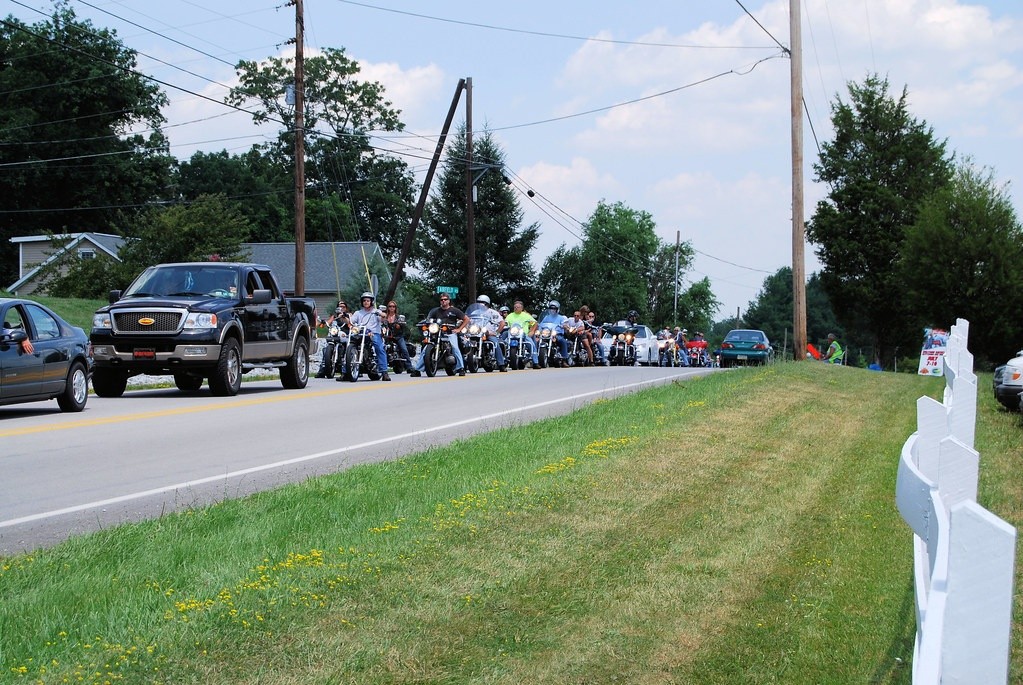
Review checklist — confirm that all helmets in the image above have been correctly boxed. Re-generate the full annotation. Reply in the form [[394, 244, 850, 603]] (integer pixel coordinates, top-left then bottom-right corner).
[[360, 292, 375, 303], [548, 300, 560, 314], [627, 310, 639, 319], [477, 295, 490, 305], [694, 331, 704, 338], [336, 300, 347, 308], [499, 306, 510, 313]]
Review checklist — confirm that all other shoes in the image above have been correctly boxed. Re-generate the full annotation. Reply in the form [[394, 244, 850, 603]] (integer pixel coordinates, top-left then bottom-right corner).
[[407, 367, 415, 373], [459, 369, 465, 376], [410, 370, 421, 377], [382, 373, 392, 381], [314, 373, 326, 379], [336, 373, 350, 381], [561, 361, 569, 368], [500, 365, 507, 372], [533, 364, 541, 369], [589, 360, 642, 366]]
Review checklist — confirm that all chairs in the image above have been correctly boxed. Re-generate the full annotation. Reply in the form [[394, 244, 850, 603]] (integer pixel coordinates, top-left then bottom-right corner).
[[830, 351, 846, 366], [732, 336, 740, 340], [752, 337, 759, 341]]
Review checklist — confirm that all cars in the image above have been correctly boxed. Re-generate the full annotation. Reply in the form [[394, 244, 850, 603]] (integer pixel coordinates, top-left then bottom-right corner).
[[720, 329, 773, 368], [601, 324, 663, 366], [0, 297, 97, 412], [993, 350, 1023, 414]]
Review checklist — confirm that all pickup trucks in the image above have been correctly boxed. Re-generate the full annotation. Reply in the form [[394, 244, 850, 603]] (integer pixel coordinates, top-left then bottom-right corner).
[[89, 262, 321, 396]]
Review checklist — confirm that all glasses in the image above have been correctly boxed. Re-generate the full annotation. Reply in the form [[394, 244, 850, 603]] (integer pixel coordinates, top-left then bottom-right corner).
[[389, 305, 396, 307], [574, 310, 594, 318], [440, 298, 448, 301], [674, 330, 679, 331], [665, 328, 670, 330], [630, 315, 636, 318]]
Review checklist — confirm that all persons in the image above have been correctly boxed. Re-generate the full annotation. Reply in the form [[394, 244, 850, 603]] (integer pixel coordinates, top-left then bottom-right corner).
[[336, 292, 391, 381], [822, 333, 843, 364], [314, 291, 353, 378], [869, 357, 883, 371], [410, 293, 469, 377], [381, 300, 414, 374], [499, 301, 541, 370], [606, 310, 641, 367], [538, 300, 569, 368], [2, 327, 34, 355], [565, 305, 606, 367], [659, 326, 706, 367], [471, 295, 507, 372]]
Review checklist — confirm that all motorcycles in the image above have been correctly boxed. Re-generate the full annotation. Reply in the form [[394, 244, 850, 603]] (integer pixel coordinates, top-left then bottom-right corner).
[[317, 312, 641, 382], [659, 338, 711, 367]]
